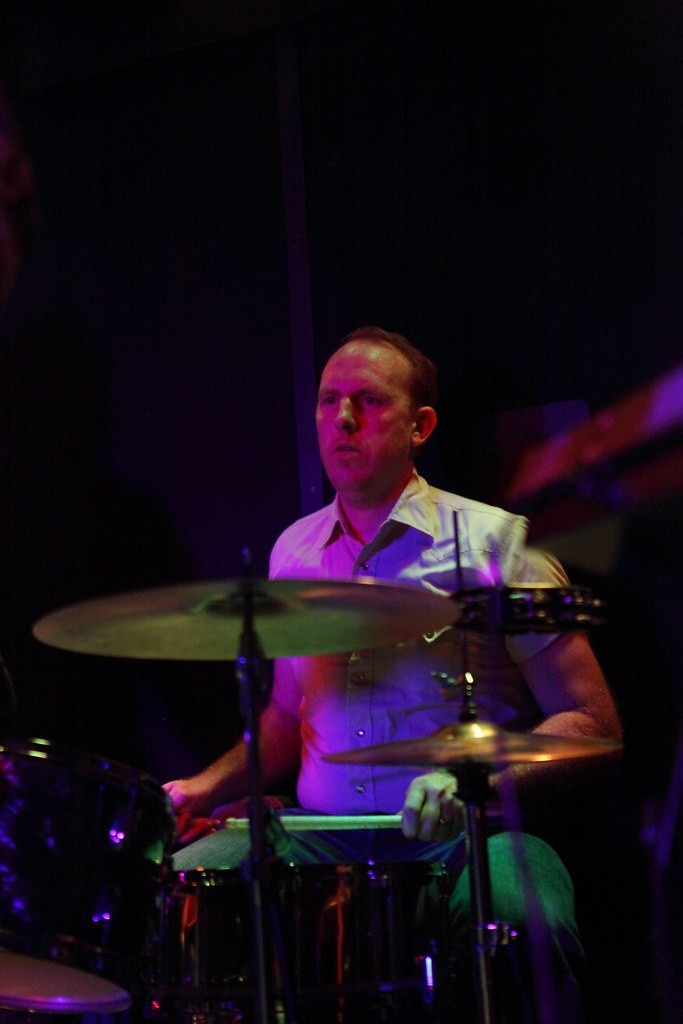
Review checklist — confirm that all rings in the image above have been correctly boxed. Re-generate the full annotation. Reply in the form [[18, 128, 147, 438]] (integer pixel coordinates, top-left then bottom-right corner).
[[438, 817, 453, 827]]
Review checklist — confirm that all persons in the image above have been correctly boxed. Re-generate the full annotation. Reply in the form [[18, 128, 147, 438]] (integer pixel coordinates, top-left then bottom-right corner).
[[159, 323, 629, 1023]]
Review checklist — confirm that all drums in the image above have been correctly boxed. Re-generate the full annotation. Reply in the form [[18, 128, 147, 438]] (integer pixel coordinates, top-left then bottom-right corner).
[[0, 736, 177, 1016], [452, 581, 610, 631], [152, 859, 451, 1024]]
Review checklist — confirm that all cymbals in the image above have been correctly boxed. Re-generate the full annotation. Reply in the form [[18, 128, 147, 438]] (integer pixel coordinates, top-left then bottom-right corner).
[[321, 718, 614, 769], [34, 576, 459, 660]]
